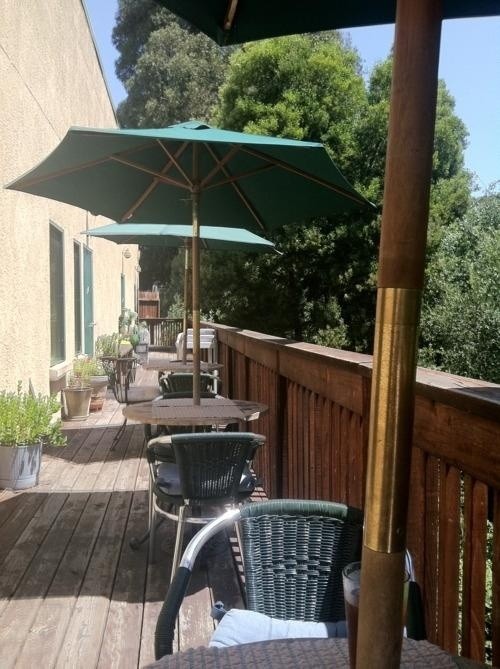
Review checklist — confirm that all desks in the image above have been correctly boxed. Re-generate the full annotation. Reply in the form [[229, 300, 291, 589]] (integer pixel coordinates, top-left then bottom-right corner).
[[140, 627, 488, 667]]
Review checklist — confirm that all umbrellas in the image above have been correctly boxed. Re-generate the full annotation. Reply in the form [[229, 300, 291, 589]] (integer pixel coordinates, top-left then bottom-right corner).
[[157, 0, 500, 667], [82, 224, 275, 366], [3, 117, 378, 406]]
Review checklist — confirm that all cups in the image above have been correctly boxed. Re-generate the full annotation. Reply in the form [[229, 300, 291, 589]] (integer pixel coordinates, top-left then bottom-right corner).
[[340, 560, 410, 669]]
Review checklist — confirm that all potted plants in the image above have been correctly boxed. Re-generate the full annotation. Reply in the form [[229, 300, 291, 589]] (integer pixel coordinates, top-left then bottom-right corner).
[[0, 383, 66, 491], [64, 359, 95, 421], [90, 359, 110, 413]]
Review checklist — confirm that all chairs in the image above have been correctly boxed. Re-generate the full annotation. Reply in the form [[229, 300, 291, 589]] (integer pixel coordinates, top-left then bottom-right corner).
[[155, 498, 427, 659]]
